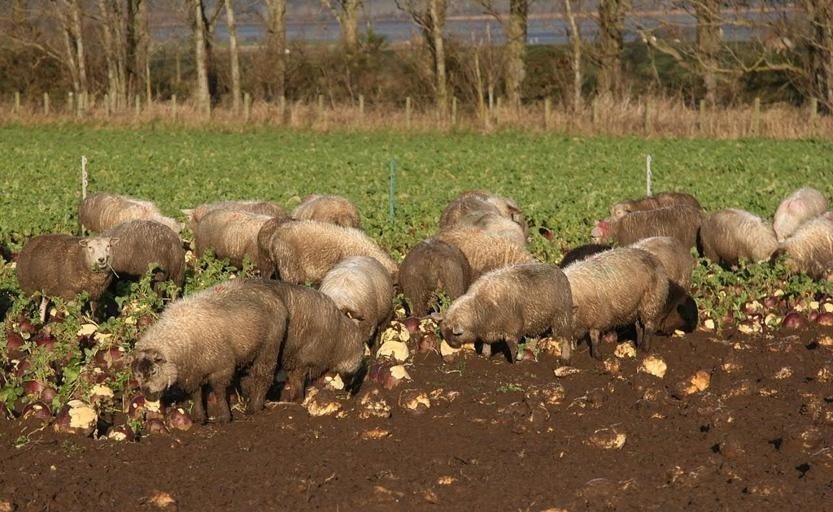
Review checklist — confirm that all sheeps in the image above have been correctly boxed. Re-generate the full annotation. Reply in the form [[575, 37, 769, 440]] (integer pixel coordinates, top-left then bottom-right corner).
[[15, 185, 833, 428]]
